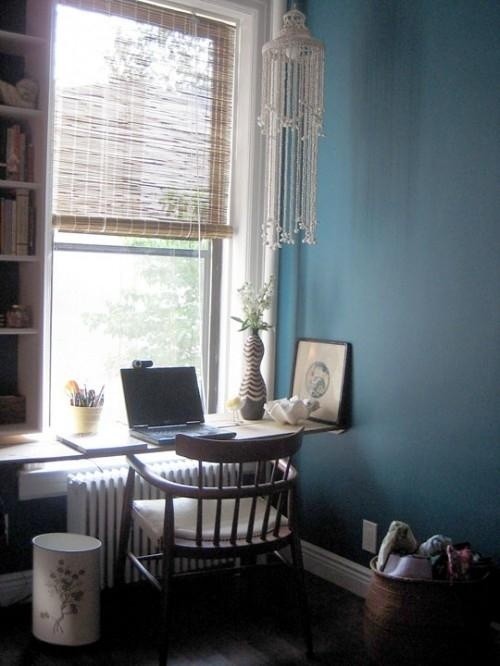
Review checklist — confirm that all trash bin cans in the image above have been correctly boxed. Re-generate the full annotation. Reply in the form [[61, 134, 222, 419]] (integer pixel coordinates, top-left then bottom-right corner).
[[31, 533, 103, 646]]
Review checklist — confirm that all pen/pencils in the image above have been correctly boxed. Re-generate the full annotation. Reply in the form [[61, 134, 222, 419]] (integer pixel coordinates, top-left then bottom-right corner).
[[64, 379, 105, 407]]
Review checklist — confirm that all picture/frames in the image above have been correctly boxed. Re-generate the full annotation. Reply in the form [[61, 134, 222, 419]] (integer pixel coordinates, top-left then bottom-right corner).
[[289, 338, 351, 425]]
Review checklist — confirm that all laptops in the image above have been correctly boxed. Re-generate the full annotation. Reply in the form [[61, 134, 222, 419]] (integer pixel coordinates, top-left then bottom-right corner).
[[119, 366, 237, 445]]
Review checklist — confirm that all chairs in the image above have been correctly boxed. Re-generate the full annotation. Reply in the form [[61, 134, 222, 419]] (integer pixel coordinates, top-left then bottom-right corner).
[[113, 426, 315, 666]]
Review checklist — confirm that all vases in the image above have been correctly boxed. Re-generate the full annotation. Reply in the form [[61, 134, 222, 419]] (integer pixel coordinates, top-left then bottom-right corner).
[[239, 329, 267, 420]]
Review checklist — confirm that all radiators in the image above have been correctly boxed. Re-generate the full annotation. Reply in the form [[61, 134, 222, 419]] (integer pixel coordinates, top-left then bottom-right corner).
[[67, 461, 258, 589]]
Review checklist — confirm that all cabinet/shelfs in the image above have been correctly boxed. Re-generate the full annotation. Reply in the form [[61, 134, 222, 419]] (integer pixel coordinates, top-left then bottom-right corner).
[[0, 1, 55, 447]]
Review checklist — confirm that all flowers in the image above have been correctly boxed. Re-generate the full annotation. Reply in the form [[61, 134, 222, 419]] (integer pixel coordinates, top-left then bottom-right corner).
[[231, 273, 278, 339]]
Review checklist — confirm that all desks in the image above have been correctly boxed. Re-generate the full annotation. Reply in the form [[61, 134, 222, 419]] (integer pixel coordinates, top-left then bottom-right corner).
[[1, 413, 348, 466]]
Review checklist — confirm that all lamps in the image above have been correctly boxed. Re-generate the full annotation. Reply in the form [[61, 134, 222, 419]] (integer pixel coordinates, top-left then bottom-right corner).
[[256, 0, 325, 250]]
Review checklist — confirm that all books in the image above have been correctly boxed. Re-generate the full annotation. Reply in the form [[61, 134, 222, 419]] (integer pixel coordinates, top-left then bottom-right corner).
[[0, 123, 36, 256], [56, 429, 148, 455]]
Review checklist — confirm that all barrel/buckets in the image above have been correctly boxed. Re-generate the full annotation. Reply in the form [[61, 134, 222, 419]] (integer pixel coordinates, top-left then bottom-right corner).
[[361, 560, 484, 664]]
